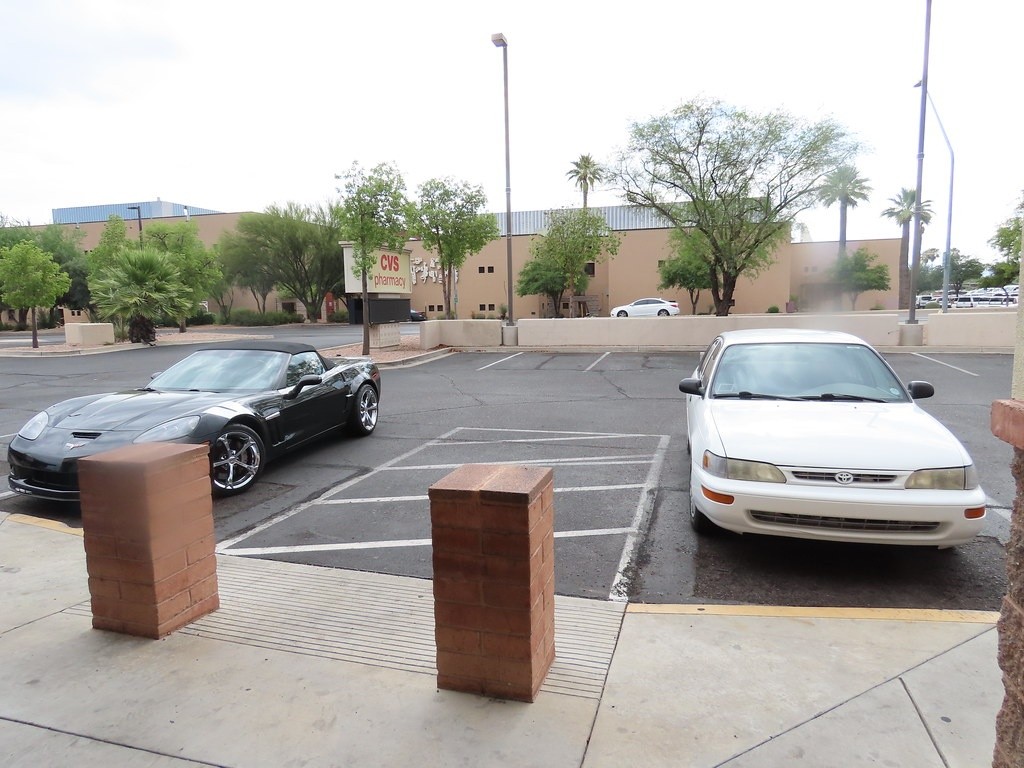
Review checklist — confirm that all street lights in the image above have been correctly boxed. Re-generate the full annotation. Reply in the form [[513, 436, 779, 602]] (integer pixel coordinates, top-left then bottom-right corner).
[[491, 32, 513, 326], [127, 206, 143, 249]]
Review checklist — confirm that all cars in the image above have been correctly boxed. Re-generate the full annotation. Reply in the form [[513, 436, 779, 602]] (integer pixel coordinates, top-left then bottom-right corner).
[[610, 297, 680, 318], [678, 327, 987, 550], [410, 309, 428, 322], [915, 284, 1020, 309]]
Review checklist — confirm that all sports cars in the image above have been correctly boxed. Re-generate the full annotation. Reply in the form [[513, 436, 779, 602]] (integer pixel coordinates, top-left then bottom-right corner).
[[7, 339, 381, 502]]
